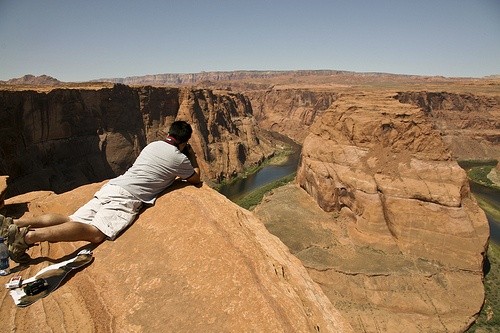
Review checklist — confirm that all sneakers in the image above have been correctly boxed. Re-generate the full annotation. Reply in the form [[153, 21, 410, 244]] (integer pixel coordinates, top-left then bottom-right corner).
[[7, 223, 31, 266], [0, 215, 15, 245]]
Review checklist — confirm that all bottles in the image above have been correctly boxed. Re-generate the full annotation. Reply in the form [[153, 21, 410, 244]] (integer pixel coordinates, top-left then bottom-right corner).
[[0, 237, 11, 276]]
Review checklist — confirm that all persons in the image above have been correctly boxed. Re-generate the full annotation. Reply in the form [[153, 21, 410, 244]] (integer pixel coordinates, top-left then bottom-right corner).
[[0, 120, 200, 264]]
